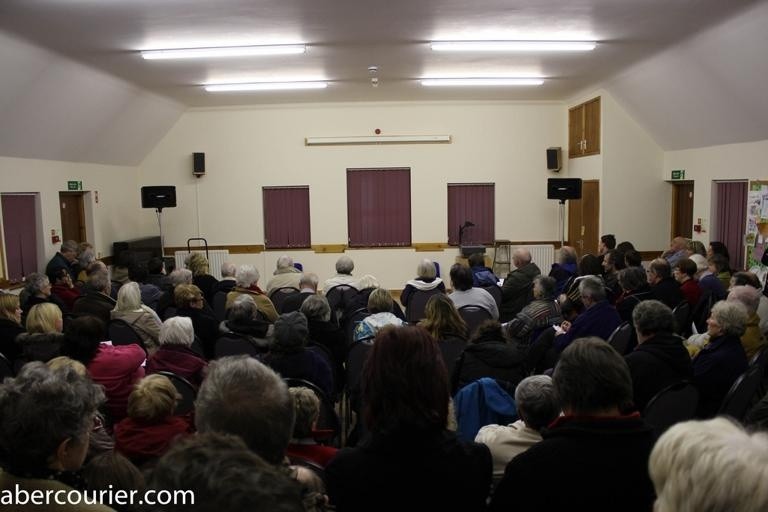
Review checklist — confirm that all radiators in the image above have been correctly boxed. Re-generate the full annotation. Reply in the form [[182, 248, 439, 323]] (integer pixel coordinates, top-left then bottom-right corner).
[[175, 249, 229, 280], [500, 244, 556, 278]]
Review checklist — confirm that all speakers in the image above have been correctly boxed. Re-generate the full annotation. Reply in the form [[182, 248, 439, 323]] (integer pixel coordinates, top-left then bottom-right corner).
[[141, 186, 176, 208], [546, 147, 561, 170], [192, 152, 205, 174], [547, 178, 582, 199]]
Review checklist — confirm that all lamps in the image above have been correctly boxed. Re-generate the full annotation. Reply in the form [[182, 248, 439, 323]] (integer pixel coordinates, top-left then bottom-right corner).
[[458, 220, 475, 246]]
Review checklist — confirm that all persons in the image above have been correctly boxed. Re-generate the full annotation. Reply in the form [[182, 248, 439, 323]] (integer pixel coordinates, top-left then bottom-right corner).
[[0, 236, 768, 512]]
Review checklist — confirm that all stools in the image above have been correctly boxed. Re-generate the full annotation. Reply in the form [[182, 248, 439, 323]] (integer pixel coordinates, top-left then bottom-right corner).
[[491, 240, 512, 273]]
